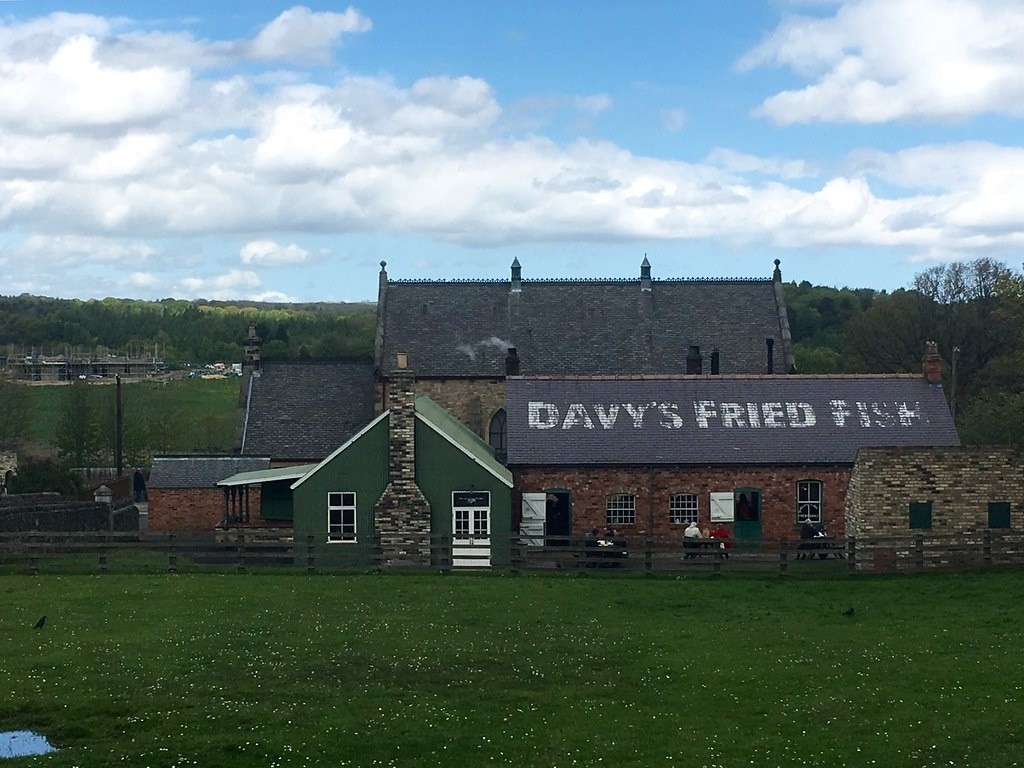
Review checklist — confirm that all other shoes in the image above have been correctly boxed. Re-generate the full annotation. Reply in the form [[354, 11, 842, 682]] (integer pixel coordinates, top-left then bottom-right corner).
[[720, 555, 724, 559], [684, 556, 688, 559], [585, 562, 622, 568], [725, 553, 729, 559]]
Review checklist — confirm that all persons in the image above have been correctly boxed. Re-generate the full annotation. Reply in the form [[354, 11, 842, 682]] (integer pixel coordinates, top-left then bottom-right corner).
[[683, 521, 703, 559], [709, 523, 733, 559], [2, 470, 20, 495], [736, 494, 752, 521], [582, 526, 629, 568], [797, 518, 820, 558]]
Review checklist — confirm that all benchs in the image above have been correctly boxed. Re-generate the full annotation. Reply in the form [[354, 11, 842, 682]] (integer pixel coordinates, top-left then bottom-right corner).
[[682, 536, 729, 560], [800, 536, 845, 561]]
